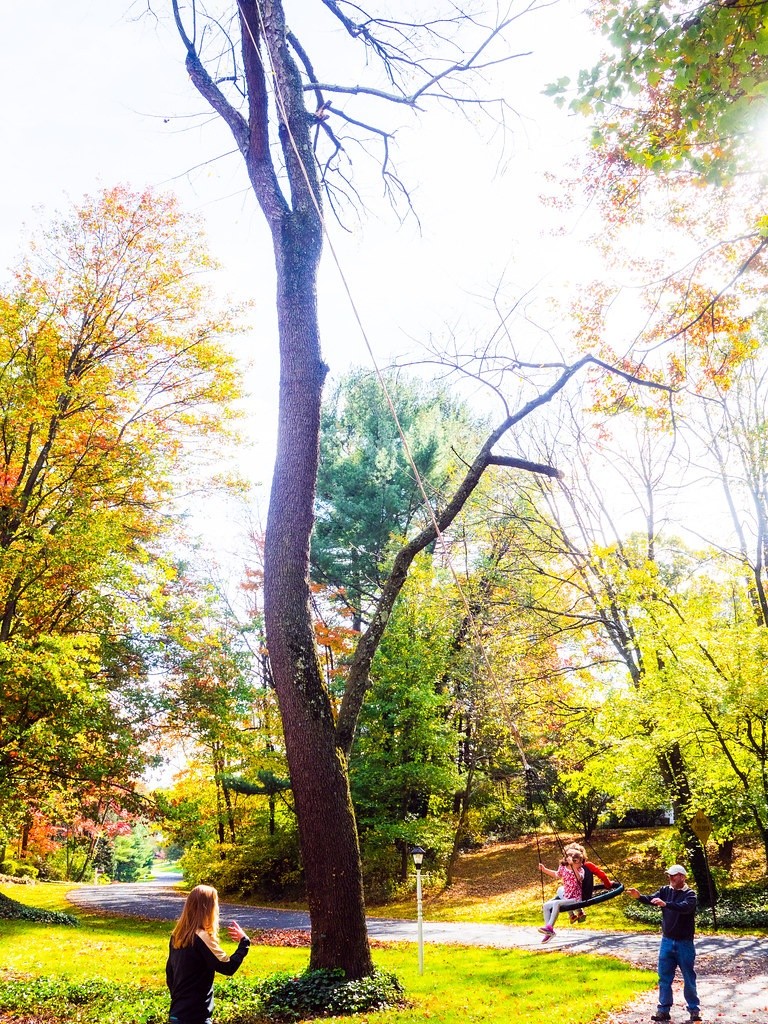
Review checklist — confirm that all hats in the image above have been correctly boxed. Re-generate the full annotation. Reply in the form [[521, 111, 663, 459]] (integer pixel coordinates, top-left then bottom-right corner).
[[664, 865, 686, 877]]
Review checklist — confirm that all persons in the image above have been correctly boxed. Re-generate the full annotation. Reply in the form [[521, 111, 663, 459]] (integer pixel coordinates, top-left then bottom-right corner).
[[538, 842, 615, 944], [166, 885, 251, 1024], [625, 864, 701, 1021]]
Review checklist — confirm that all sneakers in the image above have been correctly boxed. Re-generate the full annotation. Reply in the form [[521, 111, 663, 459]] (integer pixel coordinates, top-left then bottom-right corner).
[[538, 927, 552, 936], [651, 1011, 671, 1021], [569, 915, 577, 924], [690, 1012, 702, 1021], [577, 912, 586, 924], [542, 930, 557, 944]]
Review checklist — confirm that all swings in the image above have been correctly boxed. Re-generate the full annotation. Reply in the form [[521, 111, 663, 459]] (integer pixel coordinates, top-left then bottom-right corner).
[[524, 766, 625, 913]]
